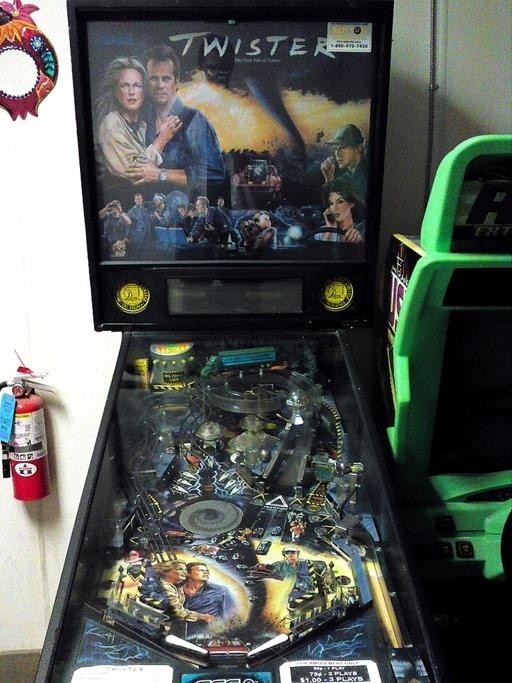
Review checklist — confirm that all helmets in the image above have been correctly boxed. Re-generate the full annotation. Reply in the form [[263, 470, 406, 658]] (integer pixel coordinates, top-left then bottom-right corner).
[[282, 547, 299, 554]]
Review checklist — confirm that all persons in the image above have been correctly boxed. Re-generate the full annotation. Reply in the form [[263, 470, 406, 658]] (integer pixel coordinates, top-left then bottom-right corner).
[[121, 542, 314, 640]]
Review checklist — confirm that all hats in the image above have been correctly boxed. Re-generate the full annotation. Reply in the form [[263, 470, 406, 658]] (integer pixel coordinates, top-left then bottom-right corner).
[[326, 123, 363, 148]]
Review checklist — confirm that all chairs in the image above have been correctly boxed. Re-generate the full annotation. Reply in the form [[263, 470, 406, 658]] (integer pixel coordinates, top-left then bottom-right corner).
[[386, 132, 512, 579]]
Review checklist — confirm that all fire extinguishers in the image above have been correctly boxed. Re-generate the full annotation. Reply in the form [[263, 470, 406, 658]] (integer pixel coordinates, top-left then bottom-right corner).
[[0, 367, 58, 501]]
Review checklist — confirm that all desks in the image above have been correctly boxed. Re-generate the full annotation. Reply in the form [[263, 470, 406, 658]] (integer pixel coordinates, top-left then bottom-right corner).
[[33, 333, 441, 683]]
